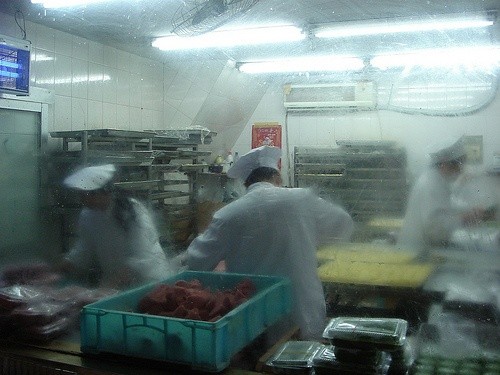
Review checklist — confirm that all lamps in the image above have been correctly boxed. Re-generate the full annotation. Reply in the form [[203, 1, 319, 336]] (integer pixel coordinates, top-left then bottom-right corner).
[[153, 6, 500, 74]]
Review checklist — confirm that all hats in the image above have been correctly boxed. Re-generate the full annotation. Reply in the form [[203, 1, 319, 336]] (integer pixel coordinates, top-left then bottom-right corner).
[[427, 138, 465, 164], [63, 163, 116, 191], [226, 145, 281, 183]]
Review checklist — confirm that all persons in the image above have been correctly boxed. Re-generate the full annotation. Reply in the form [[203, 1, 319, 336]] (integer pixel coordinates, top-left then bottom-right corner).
[[174, 149, 354, 338], [404, 137, 500, 248], [61, 163, 169, 288]]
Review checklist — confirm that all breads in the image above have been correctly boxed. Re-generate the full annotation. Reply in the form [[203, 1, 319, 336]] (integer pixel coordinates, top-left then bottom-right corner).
[[136, 280, 255, 323]]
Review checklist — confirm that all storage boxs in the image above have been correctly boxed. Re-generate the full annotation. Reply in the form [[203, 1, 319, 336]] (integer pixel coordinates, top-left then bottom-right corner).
[[81, 268, 292, 371]]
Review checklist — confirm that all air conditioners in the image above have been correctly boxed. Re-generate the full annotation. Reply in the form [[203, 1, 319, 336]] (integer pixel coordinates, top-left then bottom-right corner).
[[284, 83, 376, 112]]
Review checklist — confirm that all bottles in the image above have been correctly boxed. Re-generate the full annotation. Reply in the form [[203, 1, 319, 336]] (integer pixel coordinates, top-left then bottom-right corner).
[[199, 151, 239, 173]]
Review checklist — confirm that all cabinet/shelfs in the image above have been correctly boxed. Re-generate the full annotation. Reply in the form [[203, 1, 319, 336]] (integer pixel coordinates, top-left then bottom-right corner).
[[62, 133, 199, 257]]
